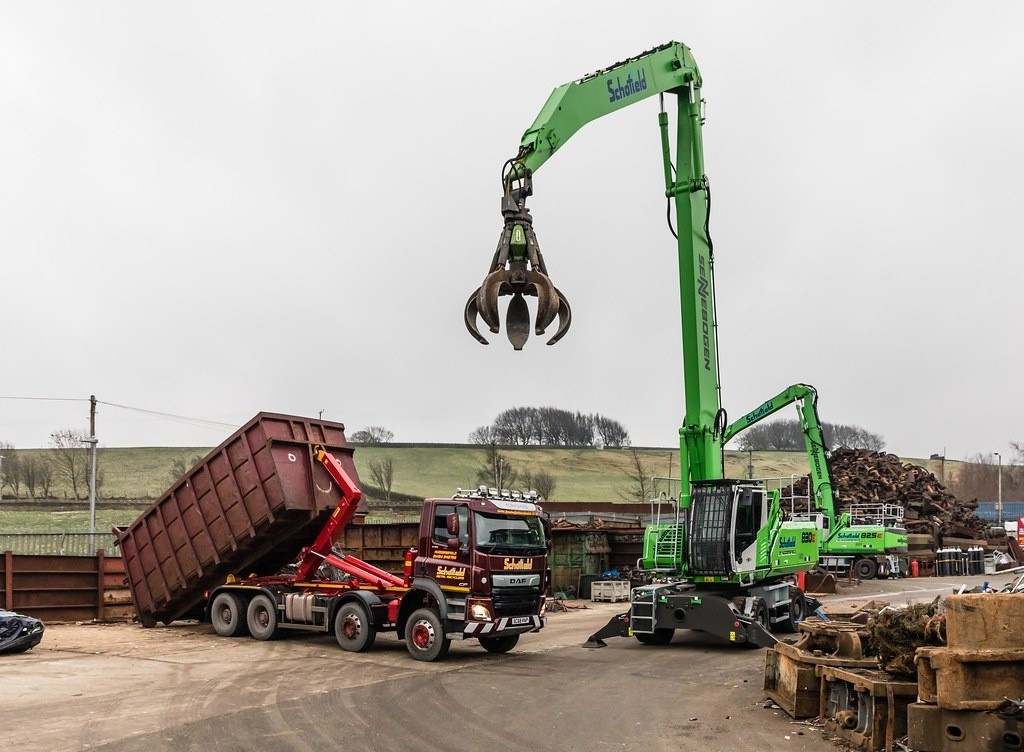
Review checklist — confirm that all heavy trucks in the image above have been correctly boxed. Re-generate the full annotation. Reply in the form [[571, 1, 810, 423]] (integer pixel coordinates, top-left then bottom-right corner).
[[112, 409, 552, 661]]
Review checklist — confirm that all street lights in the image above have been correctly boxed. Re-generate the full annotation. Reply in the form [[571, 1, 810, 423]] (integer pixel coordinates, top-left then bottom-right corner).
[[993, 453, 1001, 525]]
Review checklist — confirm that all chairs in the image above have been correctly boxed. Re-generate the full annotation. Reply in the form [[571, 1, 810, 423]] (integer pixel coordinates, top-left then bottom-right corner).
[[735, 505, 752, 537]]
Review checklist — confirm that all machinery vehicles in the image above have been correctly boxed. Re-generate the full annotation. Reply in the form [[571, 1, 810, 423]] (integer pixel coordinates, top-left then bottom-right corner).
[[723, 384, 909, 581], [462, 39, 852, 650]]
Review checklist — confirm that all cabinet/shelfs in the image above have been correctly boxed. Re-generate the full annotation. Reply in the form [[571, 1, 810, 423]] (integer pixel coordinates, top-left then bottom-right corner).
[[591, 581, 630, 602]]
